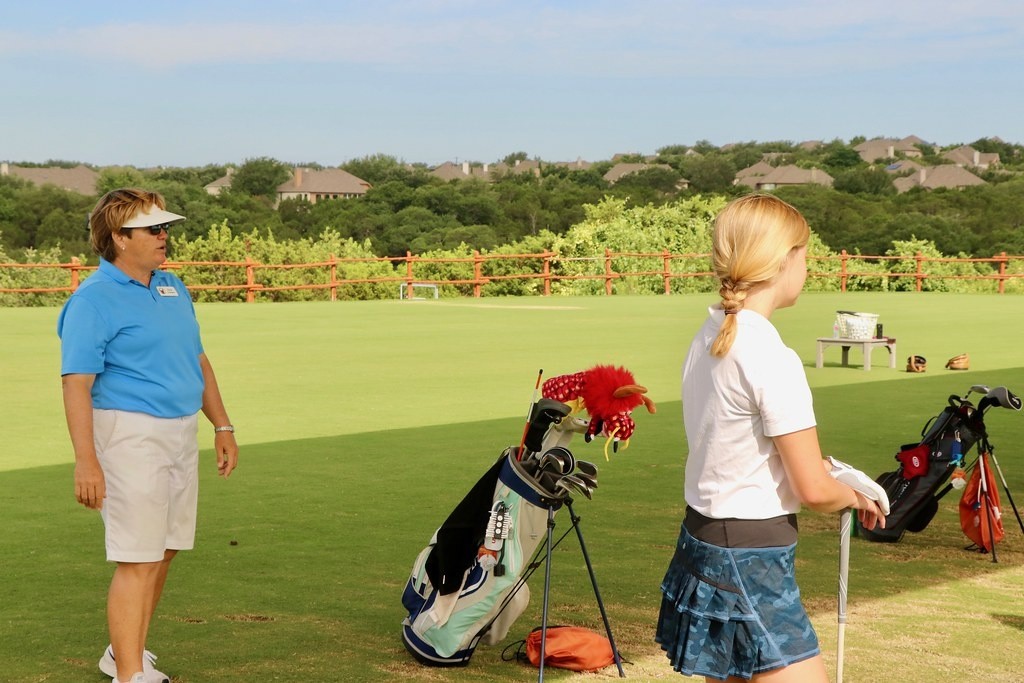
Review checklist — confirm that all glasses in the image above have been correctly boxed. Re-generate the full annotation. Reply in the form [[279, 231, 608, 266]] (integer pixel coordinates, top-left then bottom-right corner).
[[127, 223, 170, 235]]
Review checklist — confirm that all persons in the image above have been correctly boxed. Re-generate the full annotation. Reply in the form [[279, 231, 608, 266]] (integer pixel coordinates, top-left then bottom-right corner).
[[57, 188, 239, 683], [654, 195, 890, 683]]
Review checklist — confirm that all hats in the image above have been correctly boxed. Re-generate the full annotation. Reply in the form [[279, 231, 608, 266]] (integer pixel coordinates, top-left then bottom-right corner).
[[122, 204, 185, 228]]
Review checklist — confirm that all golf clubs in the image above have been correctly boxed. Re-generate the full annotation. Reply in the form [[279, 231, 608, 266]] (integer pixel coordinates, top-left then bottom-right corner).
[[948, 384, 990, 413], [835, 506, 855, 683], [553, 459, 600, 501]]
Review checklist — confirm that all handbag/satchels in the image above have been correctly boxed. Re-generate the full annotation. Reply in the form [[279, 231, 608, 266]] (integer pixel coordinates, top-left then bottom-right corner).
[[907, 356, 926, 372], [502, 625, 620, 674], [826, 455, 890, 516], [946, 353, 968, 370], [959, 436, 1004, 554]]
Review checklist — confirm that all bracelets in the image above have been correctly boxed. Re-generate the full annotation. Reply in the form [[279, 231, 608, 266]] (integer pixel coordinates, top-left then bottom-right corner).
[[215, 425, 234, 433]]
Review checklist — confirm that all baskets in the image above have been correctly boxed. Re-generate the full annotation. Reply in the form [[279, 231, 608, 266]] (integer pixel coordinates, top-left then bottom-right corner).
[[846, 317, 869, 340], [838, 311, 879, 340]]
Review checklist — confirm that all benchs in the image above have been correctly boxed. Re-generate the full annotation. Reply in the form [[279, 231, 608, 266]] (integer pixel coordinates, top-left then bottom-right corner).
[[400, 284, 439, 298]]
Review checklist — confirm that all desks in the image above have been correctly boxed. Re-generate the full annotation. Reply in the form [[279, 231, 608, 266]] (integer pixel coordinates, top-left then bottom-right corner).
[[816, 337, 897, 371]]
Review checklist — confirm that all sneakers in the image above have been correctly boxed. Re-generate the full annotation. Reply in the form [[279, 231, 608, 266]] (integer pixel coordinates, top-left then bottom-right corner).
[[99, 643, 170, 683], [112, 671, 149, 683]]
